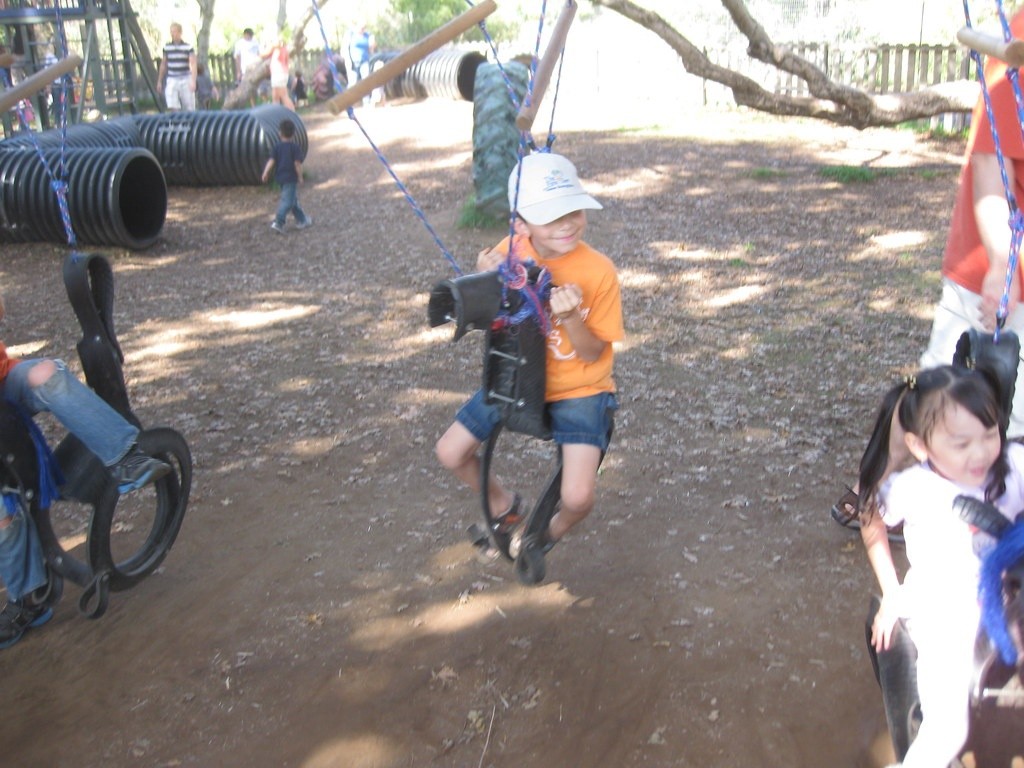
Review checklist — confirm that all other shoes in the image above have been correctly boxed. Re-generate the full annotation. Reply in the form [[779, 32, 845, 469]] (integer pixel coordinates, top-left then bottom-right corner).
[[295, 214, 313, 230], [271, 220, 289, 236]]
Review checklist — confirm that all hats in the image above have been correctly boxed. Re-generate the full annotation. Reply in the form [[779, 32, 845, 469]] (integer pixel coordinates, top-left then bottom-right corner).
[[506, 152, 603, 227]]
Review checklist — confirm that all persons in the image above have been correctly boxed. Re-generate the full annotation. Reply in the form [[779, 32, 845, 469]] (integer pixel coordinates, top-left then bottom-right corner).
[[262, 120, 313, 233], [857, 367, 1024, 768], [235, 28, 296, 113], [195, 65, 219, 111], [156, 23, 198, 112], [1, 339, 170, 649], [434, 153, 624, 559], [1, 30, 61, 130], [291, 71, 308, 109], [925, 5, 1024, 439], [344, 24, 373, 108]]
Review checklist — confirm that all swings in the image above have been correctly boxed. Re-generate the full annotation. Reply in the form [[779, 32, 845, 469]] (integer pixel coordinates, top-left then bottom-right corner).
[[862, 0, 1023, 768], [0, 1, 196, 619], [312, 0, 617, 587]]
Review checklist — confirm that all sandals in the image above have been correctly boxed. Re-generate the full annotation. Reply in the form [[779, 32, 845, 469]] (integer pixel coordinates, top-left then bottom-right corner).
[[476, 491, 529, 562], [508, 508, 570, 561], [829, 482, 905, 544]]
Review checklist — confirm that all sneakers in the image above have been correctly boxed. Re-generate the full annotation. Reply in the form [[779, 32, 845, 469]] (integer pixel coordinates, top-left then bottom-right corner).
[[106, 437, 173, 496], [0, 591, 52, 649]]
